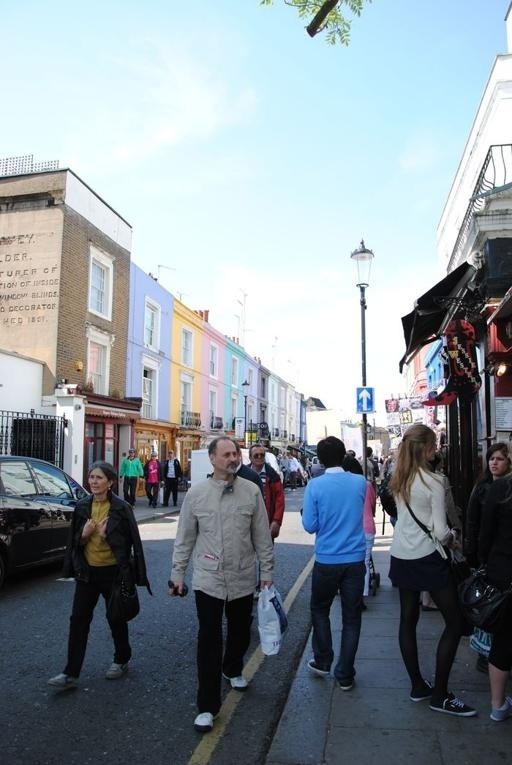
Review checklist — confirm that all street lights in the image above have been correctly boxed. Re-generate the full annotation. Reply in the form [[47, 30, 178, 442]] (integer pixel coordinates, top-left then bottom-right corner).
[[240, 378, 251, 443], [349, 238, 377, 490]]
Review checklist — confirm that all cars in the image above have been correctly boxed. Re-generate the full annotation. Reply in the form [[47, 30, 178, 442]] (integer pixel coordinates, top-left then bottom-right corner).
[[278, 455, 325, 489], [0, 453, 91, 583]]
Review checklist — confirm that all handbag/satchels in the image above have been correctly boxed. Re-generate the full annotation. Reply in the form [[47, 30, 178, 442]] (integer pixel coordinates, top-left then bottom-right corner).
[[377, 472, 397, 520], [106, 567, 140, 625], [455, 563, 512, 629], [256, 584, 290, 656]]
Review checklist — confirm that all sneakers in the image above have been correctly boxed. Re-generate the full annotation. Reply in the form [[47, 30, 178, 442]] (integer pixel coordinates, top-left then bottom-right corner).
[[410, 679, 435, 702], [490, 695, 512, 722], [335, 672, 354, 690], [222, 669, 249, 690], [308, 657, 330, 675], [194, 710, 214, 731], [429, 689, 477, 717], [104, 663, 129, 679], [48, 672, 81, 689]]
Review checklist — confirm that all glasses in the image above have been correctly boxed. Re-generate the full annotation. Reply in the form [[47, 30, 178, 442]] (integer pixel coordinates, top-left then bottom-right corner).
[[252, 453, 264, 459]]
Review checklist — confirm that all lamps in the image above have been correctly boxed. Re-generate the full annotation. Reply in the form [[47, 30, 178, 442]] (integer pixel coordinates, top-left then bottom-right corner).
[[74, 360, 84, 372]]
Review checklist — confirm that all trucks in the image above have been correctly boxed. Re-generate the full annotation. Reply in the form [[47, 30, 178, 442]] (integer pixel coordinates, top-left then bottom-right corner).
[[188, 447, 286, 513]]
